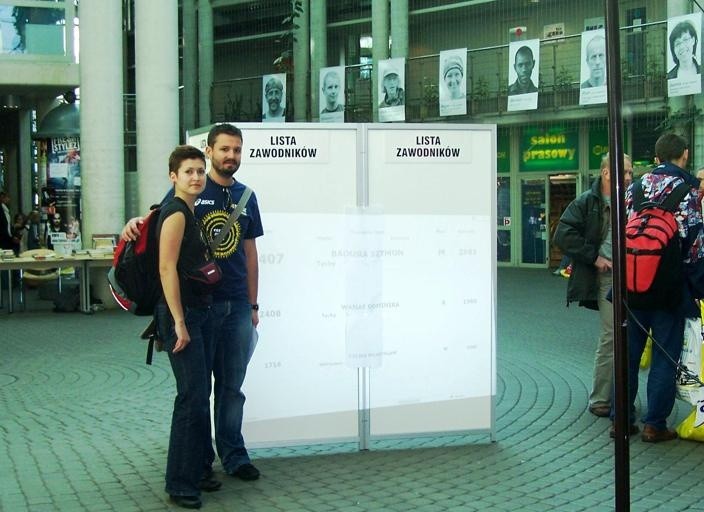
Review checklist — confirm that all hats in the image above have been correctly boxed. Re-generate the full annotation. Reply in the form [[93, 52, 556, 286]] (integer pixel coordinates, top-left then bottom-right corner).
[[265, 78, 283, 92], [383, 66, 399, 78], [442, 55, 464, 79]]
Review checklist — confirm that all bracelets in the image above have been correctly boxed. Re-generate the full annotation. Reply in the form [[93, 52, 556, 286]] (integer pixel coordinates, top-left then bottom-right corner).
[[251, 304, 259, 310]]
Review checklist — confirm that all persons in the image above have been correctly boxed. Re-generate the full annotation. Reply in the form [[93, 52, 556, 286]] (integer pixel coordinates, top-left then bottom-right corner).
[[439, 55, 465, 100], [605, 134, 704, 444], [122, 125, 264, 481], [581, 35, 607, 88], [0, 192, 68, 258], [666, 21, 701, 80], [153, 145, 221, 509], [379, 65, 405, 108], [508, 46, 538, 95], [554, 152, 633, 418], [321, 71, 344, 114], [262, 78, 286, 119]]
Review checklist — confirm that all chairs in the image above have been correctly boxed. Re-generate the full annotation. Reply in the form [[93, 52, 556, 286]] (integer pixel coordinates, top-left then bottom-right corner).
[[19, 249, 61, 311]]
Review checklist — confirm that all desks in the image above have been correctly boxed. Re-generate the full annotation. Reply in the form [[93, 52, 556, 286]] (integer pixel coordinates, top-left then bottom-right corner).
[[87, 256, 115, 313], [0, 255, 86, 314]]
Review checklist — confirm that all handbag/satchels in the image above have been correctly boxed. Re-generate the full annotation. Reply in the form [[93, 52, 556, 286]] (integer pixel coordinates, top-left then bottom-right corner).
[[183, 257, 225, 296]]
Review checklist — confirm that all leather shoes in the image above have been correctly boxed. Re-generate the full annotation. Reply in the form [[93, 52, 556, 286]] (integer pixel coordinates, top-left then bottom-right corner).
[[589, 407, 610, 417], [610, 423, 639, 438], [169, 494, 201, 509], [641, 425, 678, 443], [237, 463, 259, 481], [204, 479, 221, 493]]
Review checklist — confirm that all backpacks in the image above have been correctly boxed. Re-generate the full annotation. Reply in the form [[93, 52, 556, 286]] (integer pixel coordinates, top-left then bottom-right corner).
[[625, 177, 698, 312], [107, 200, 193, 366]]
